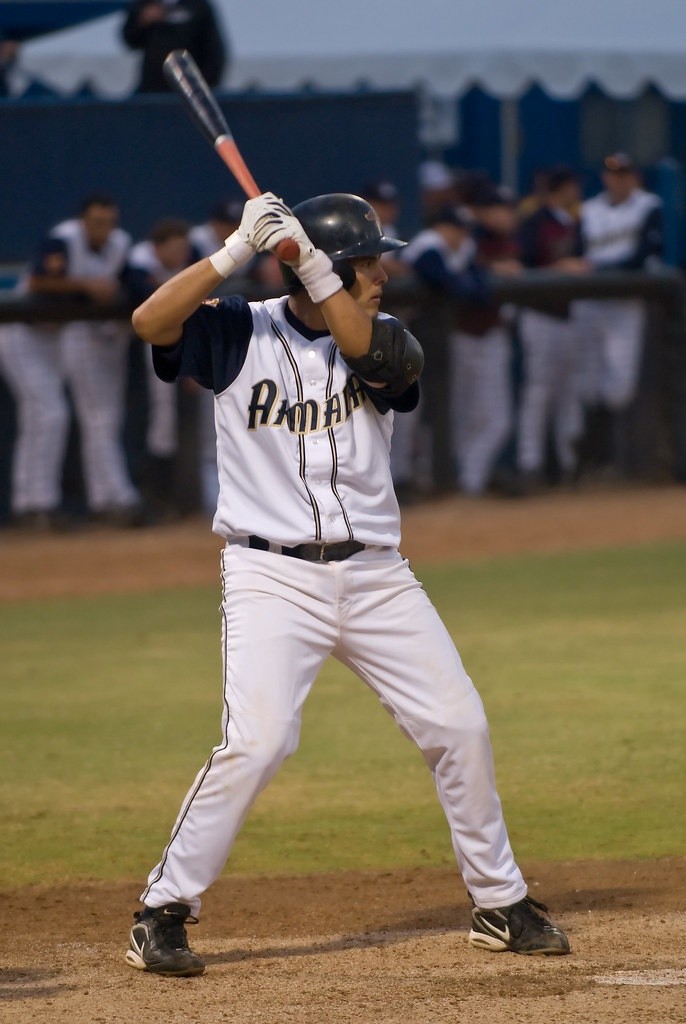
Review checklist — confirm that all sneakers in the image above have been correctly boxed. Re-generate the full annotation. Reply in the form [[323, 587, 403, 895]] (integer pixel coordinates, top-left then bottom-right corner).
[[464, 889, 570, 954], [123, 904, 205, 977]]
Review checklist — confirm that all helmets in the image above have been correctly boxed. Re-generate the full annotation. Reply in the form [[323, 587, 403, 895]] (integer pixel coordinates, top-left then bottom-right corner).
[[277, 192, 408, 293]]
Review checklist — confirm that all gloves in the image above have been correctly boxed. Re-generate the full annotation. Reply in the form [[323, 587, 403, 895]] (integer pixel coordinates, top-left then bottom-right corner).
[[210, 192, 294, 279], [246, 208, 343, 303]]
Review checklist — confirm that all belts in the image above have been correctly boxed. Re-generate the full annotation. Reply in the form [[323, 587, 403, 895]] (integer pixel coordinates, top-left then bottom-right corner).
[[249, 535, 365, 565]]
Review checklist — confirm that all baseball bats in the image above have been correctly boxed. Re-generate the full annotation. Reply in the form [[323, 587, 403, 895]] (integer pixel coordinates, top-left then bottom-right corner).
[[162, 48, 300, 262]]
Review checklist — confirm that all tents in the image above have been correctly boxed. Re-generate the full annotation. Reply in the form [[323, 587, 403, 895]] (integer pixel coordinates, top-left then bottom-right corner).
[[1, 1, 686, 323]]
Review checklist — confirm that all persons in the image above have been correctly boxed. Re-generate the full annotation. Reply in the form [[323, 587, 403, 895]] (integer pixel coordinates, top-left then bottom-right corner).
[[0, 155, 662, 525], [124, 196, 570, 978]]
